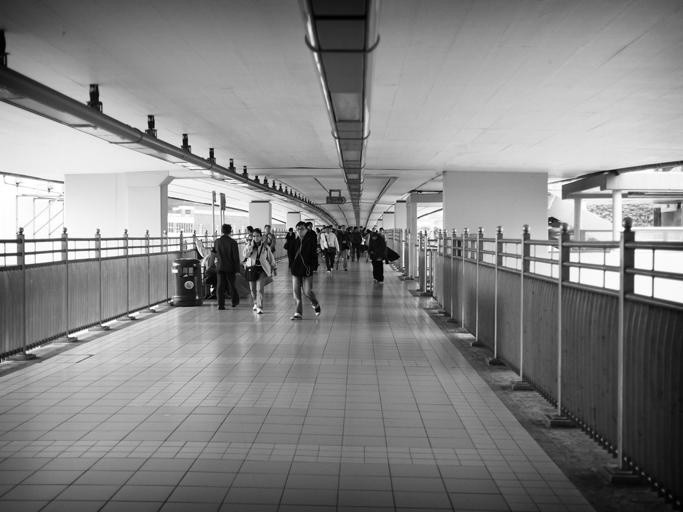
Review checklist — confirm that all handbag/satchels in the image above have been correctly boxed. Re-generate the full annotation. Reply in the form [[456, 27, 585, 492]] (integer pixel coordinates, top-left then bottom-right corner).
[[245, 265, 260, 280], [328, 247, 336, 254]]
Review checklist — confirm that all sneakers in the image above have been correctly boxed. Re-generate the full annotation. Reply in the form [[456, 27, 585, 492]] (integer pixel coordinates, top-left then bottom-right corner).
[[253, 304, 264, 314], [290, 306, 321, 320]]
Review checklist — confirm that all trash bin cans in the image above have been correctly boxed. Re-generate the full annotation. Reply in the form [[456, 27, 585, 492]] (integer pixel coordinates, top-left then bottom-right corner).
[[170, 258, 203, 306]]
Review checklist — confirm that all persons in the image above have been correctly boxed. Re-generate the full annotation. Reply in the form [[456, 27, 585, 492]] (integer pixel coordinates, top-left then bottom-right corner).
[[244, 223, 400, 286], [212, 223, 241, 311], [241, 227, 277, 316], [200, 248, 221, 299], [288, 221, 321, 321]]
